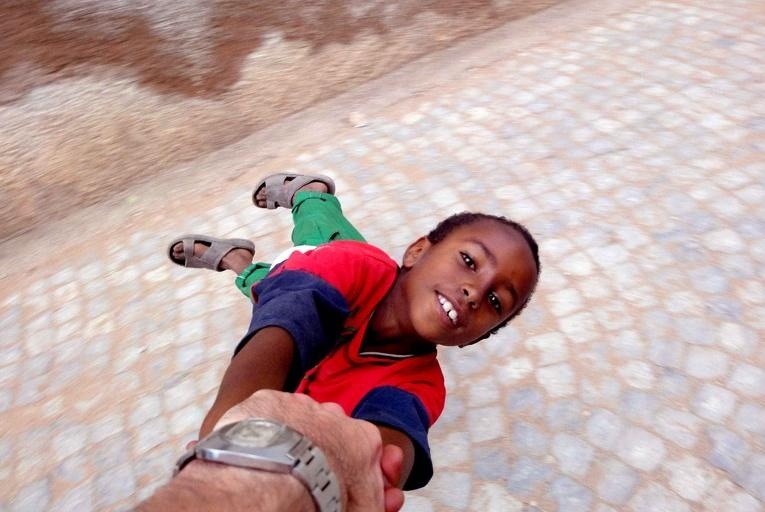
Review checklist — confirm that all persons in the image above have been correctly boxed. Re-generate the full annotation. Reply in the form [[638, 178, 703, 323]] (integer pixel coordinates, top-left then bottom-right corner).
[[166, 167, 543, 512], [121, 386, 394, 512]]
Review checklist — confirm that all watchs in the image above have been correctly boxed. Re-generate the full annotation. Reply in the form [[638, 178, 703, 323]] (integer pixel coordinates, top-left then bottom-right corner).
[[169, 415, 345, 512]]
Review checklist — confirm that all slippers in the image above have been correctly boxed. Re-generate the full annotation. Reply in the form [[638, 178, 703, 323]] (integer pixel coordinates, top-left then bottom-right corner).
[[252, 172, 336, 210], [167, 235, 256, 273]]
